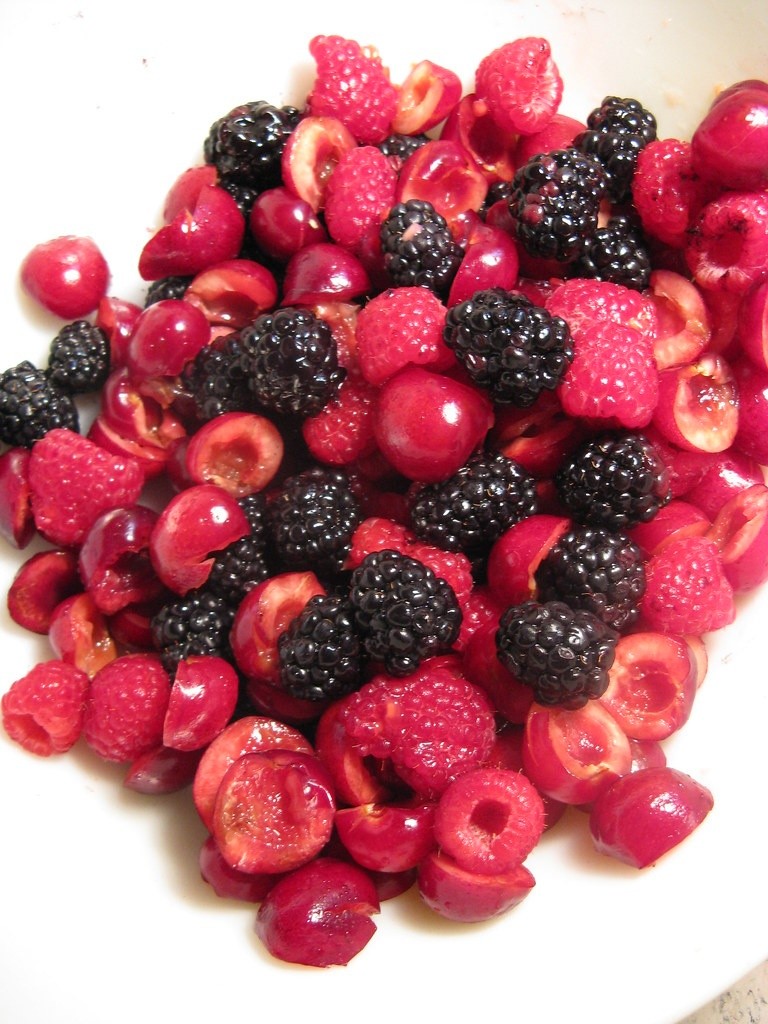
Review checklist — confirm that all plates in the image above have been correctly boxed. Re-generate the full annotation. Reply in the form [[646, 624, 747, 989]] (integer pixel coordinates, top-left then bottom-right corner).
[[3, 2, 768, 1023]]
